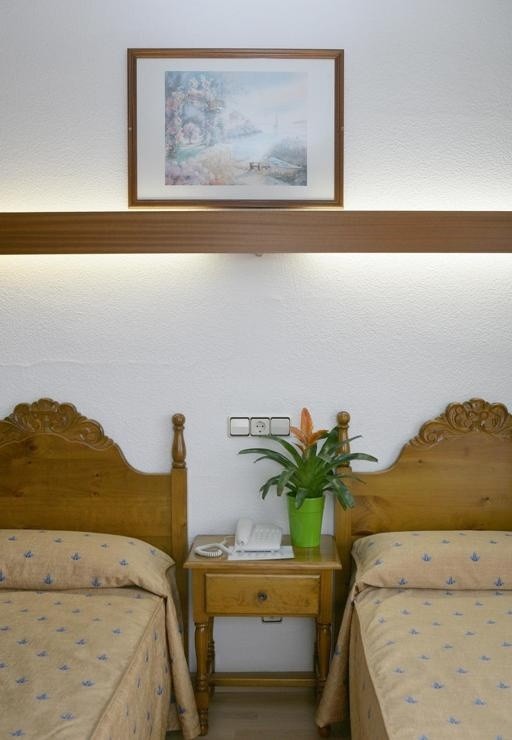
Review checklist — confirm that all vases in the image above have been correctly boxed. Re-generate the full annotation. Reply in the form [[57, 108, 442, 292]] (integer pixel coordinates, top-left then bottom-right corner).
[[287, 491, 326, 547]]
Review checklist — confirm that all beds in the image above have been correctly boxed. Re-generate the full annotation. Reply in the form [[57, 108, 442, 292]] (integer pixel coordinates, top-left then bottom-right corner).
[[0, 396, 188, 740], [336, 398, 512, 740]]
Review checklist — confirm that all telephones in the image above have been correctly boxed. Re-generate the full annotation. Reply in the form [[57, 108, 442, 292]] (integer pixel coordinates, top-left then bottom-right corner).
[[235, 519, 282, 551]]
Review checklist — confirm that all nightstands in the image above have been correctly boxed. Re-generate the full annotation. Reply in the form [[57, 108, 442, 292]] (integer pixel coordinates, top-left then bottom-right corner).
[[183, 535, 343, 736]]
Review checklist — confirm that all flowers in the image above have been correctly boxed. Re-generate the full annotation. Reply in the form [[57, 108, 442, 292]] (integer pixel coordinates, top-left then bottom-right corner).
[[237, 408, 378, 511]]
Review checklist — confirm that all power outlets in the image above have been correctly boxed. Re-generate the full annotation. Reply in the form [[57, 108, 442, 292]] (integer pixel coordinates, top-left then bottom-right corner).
[[250, 417, 270, 438]]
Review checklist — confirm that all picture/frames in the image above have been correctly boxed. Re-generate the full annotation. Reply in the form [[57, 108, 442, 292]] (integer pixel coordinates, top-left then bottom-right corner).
[[125, 47, 345, 209]]
